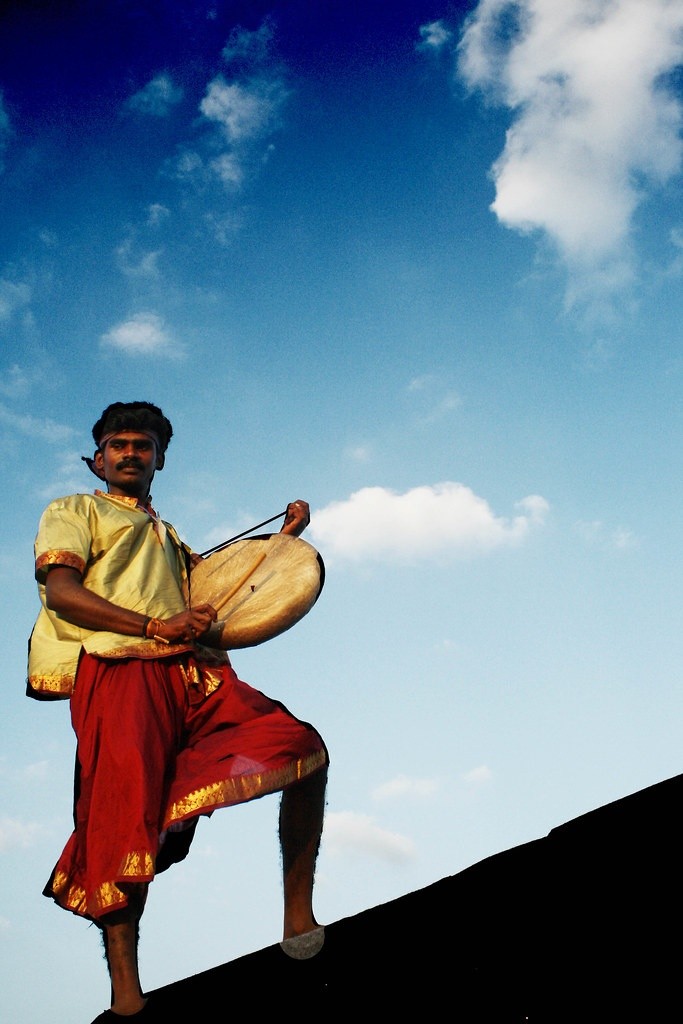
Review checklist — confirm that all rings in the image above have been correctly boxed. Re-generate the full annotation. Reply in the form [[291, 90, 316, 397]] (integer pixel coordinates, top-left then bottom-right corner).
[[295, 503, 301, 506]]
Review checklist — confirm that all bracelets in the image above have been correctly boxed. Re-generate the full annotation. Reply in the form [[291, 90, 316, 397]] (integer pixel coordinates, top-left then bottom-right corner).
[[143, 617, 170, 644]]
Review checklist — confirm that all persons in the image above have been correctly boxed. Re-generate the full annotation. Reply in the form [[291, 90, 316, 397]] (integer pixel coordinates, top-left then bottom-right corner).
[[27, 401, 330, 1023]]
